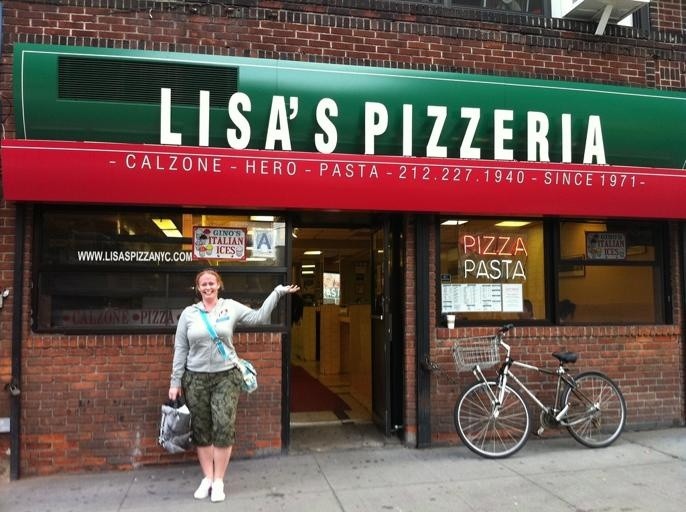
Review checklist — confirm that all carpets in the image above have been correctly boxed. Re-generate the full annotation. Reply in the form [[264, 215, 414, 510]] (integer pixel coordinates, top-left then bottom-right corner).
[[291, 364, 352, 413]]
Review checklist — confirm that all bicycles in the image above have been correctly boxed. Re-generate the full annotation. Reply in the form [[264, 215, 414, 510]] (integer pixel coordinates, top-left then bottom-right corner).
[[449, 322, 626, 459]]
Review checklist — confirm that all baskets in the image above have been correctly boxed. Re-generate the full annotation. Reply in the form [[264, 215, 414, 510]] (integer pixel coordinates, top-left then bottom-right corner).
[[451, 335, 501, 373]]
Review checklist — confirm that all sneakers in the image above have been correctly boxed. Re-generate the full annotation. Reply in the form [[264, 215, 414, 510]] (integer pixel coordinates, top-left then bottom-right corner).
[[194, 476, 212, 499], [211, 483, 225, 501]]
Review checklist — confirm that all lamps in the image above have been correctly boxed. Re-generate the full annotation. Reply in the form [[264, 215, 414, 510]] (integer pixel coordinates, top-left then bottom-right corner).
[[292, 228, 305, 238]]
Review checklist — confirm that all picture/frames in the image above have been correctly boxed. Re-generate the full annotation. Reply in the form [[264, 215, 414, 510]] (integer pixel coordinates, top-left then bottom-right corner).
[[559, 253, 585, 278], [627, 245, 647, 256]]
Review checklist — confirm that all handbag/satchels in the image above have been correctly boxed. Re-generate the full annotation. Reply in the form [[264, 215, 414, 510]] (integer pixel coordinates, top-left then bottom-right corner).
[[159, 397, 194, 455], [231, 358, 258, 394]]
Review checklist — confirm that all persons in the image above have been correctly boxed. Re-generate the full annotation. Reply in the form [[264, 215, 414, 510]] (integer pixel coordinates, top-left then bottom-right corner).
[[517, 299, 538, 320], [167, 268, 301, 501], [559, 298, 577, 323]]
